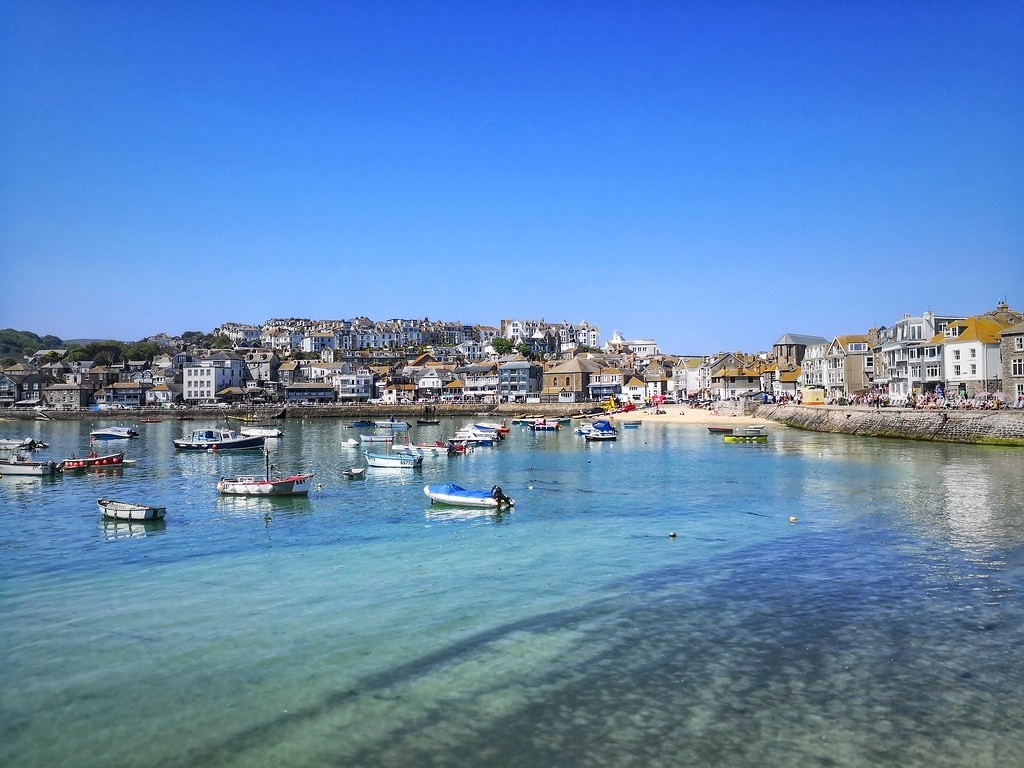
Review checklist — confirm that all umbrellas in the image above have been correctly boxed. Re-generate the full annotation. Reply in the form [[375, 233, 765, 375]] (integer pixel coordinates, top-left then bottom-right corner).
[[976, 391, 991, 404], [991, 392, 1009, 410]]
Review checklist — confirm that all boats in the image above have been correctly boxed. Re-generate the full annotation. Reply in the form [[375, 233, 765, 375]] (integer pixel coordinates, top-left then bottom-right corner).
[[90, 425, 140, 440], [423, 482, 516, 513], [171, 410, 270, 453], [240, 424, 286, 438], [0, 435, 51, 460], [177, 415, 194, 420], [140, 417, 162, 423], [227, 413, 262, 422], [342, 402, 667, 480], [216, 447, 316, 497], [62, 450, 127, 470], [97, 497, 167, 521], [0, 446, 63, 476], [709, 423, 767, 444]]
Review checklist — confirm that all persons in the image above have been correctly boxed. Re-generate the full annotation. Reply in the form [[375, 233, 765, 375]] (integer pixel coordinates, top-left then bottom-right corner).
[[825, 389, 1009, 410], [493, 486, 514, 513], [776, 392, 803, 405]]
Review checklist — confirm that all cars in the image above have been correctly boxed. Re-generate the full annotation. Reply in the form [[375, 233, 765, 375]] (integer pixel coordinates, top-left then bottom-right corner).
[[33, 404, 46, 412], [161, 401, 311, 409], [88, 403, 134, 412]]
